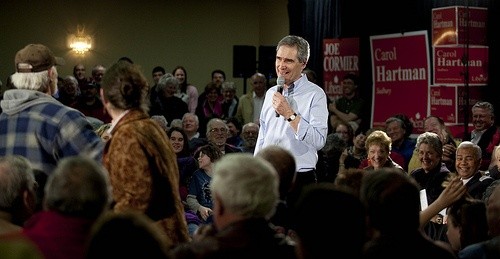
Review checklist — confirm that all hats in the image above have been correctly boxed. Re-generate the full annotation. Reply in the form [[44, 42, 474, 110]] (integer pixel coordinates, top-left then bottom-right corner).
[[15, 43, 66, 73]]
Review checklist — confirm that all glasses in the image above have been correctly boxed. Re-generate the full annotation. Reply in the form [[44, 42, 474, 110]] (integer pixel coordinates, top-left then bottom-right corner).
[[208, 129, 228, 133]]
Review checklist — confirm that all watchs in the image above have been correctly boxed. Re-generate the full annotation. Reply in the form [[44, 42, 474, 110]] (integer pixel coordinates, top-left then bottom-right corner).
[[287, 112, 298, 121]]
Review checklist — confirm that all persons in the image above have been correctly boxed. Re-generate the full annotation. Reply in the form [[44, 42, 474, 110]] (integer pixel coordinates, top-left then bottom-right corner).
[[253, 35, 329, 188], [0, 45, 500, 259]]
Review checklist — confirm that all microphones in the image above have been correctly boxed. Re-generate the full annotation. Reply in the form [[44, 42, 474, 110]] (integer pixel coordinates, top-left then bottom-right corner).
[[275, 76, 284, 116]]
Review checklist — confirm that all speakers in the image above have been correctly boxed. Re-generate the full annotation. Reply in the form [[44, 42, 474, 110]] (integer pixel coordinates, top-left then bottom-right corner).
[[232, 44, 258, 77], [259, 44, 278, 78]]
